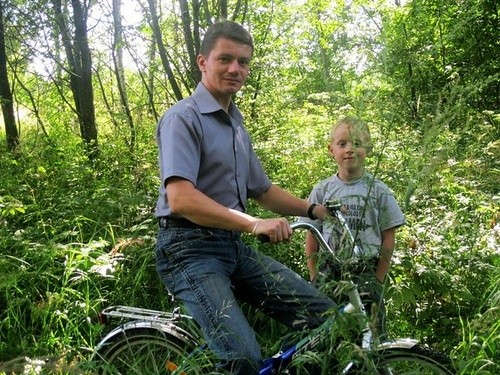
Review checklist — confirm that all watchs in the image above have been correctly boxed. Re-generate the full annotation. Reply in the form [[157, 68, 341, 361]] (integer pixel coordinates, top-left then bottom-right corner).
[[308, 203, 321, 220]]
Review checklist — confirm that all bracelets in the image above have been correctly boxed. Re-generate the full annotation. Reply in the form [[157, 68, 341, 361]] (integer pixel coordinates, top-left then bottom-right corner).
[[252, 219, 260, 237]]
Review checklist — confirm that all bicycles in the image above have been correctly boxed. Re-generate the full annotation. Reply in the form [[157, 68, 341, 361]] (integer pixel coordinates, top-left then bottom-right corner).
[[88, 198, 457, 375]]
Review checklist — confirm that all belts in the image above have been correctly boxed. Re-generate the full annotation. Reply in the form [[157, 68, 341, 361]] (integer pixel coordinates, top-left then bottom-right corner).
[[159, 216, 206, 228]]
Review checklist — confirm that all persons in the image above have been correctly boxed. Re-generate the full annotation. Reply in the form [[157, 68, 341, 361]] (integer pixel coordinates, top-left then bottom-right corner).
[[152, 21, 345, 375], [305, 119, 405, 375]]
